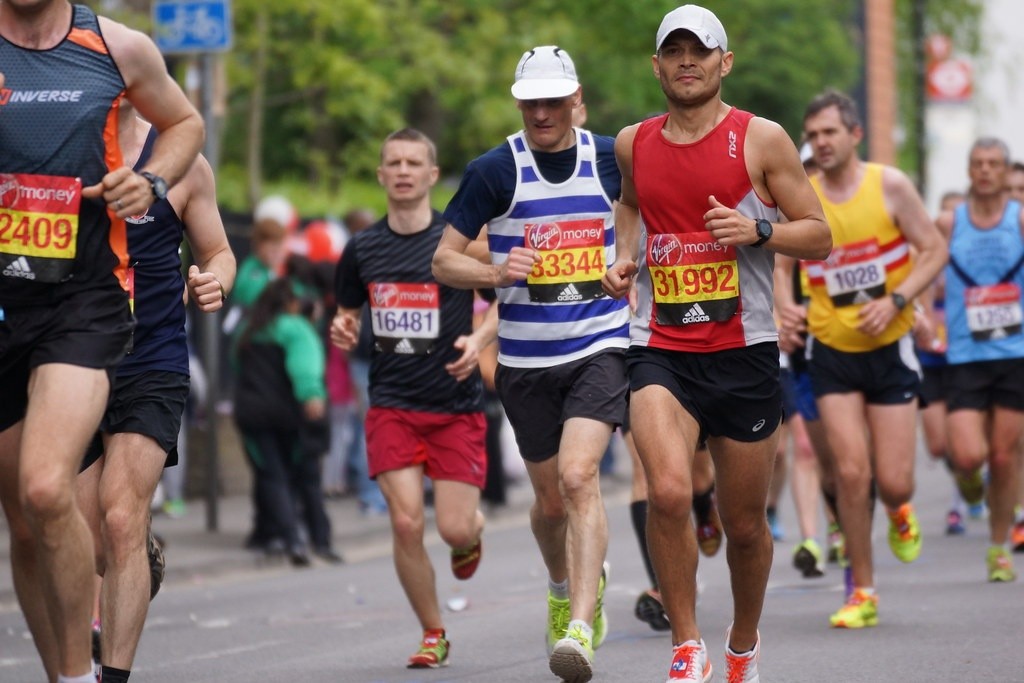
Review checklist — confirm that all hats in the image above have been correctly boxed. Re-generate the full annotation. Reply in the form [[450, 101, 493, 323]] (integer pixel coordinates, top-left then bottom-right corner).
[[510, 45, 580, 100], [656, 4, 728, 54], [800, 141, 813, 165], [255, 194, 296, 233]]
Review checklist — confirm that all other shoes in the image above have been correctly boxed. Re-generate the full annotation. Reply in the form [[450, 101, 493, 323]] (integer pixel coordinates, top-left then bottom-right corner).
[[247, 534, 310, 569], [313, 544, 341, 563]]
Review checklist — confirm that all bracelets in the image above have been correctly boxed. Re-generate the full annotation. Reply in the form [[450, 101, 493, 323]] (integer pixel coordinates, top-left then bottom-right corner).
[[211, 277, 228, 312]]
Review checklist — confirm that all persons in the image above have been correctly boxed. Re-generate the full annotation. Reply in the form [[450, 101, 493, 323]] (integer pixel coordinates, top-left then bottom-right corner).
[[0, 0, 205, 683], [599, 5, 834, 683], [72, 88, 238, 683], [429, 43, 634, 683], [330, 125, 503, 671], [219, 192, 378, 570], [620, 89, 1024, 630]]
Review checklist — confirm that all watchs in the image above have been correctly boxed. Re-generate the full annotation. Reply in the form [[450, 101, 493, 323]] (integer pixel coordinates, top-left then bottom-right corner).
[[890, 293, 907, 309], [140, 171, 169, 200], [749, 218, 773, 248]]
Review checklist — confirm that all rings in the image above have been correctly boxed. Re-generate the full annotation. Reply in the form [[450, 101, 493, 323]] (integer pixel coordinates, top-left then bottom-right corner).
[[116, 199, 126, 210]]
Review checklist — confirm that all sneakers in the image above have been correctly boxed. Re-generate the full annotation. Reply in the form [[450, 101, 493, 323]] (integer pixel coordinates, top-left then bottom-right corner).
[[91, 614, 102, 665], [883, 503, 923, 564], [766, 514, 784, 540], [830, 587, 879, 629], [635, 591, 671, 632], [946, 510, 966, 535], [957, 467, 984, 504], [1008, 519, 1024, 549], [407, 635, 450, 668], [986, 546, 1017, 582], [692, 481, 724, 557], [547, 590, 571, 654], [968, 504, 983, 515], [451, 536, 482, 580], [593, 561, 610, 650], [666, 637, 713, 683], [549, 624, 593, 683], [827, 524, 848, 562], [724, 620, 761, 683], [792, 540, 825, 578]]
